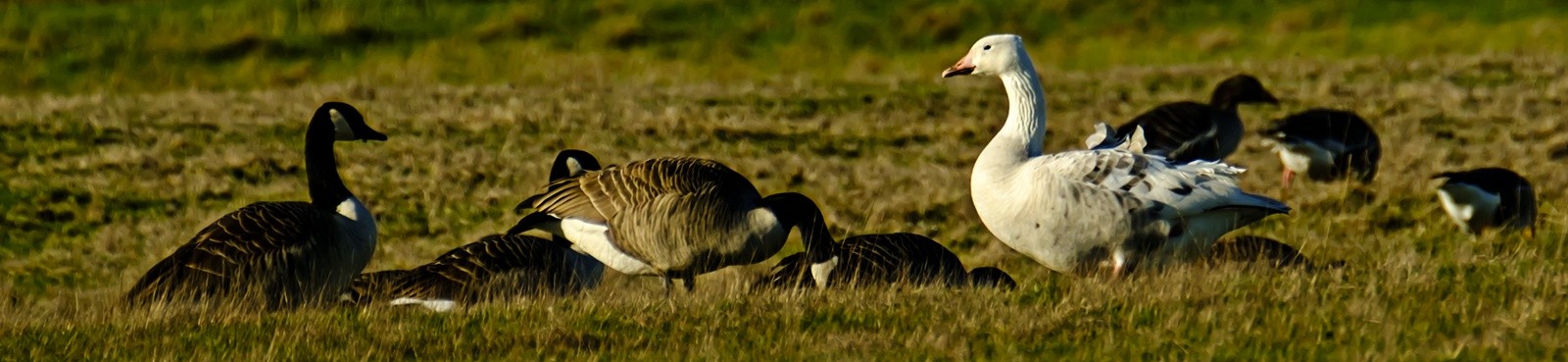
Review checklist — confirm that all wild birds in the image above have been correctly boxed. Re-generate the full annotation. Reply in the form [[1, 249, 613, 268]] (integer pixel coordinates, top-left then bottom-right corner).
[[1428, 167, 1539, 251], [117, 101, 388, 315], [510, 156, 840, 294], [1257, 106, 1383, 186], [1095, 73, 1281, 168], [342, 149, 609, 314], [750, 233, 1017, 293]]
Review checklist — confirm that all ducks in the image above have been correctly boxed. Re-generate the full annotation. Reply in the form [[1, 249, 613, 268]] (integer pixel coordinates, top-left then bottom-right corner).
[[941, 34, 1291, 285]]
